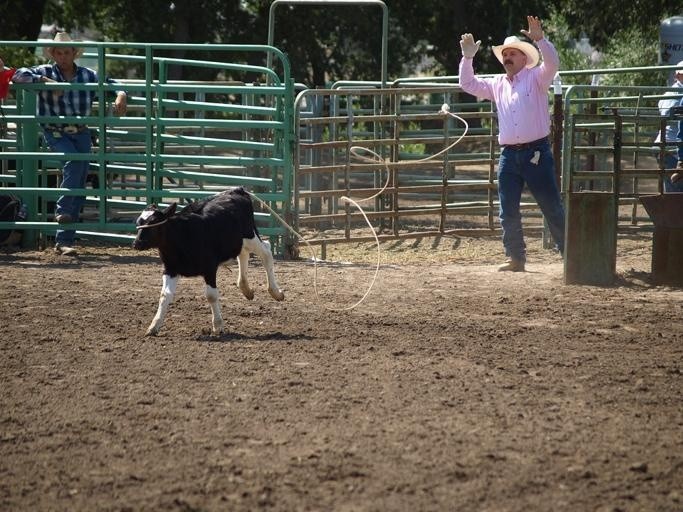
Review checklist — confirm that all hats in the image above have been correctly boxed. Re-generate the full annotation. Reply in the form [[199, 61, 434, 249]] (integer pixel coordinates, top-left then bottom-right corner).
[[492, 36, 539, 69], [675, 61, 683, 75], [42, 32, 84, 60]]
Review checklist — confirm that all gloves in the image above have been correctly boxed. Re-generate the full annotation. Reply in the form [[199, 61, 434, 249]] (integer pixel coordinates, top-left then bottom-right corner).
[[459, 33, 481, 57]]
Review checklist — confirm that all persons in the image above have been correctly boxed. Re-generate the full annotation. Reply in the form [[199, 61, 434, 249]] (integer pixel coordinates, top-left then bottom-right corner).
[[12, 32, 126, 254], [459, 16, 565, 272], [653, 61, 683, 192]]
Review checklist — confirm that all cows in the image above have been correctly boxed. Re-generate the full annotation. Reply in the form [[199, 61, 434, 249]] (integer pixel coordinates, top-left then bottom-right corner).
[[131, 188, 284, 340]]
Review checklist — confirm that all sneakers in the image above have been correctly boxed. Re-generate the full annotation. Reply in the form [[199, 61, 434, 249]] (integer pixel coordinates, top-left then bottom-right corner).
[[56, 214, 72, 222], [53, 243, 77, 256], [671, 161, 683, 183], [498, 261, 524, 272]]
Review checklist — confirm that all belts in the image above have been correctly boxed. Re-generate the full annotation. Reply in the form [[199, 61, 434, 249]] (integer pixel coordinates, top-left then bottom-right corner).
[[506, 136, 548, 150]]
